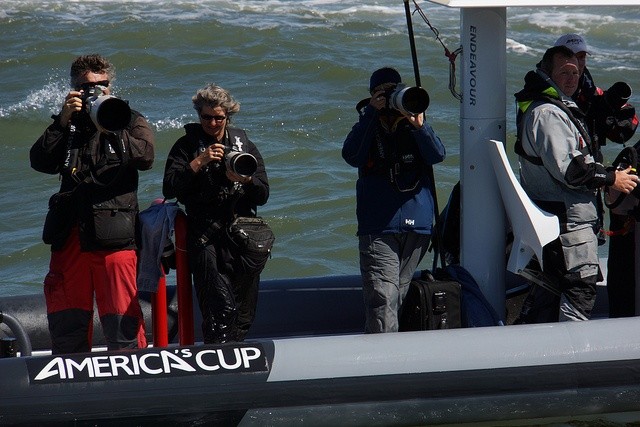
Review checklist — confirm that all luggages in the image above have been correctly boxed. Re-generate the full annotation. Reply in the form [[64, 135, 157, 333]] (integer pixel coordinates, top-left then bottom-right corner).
[[399, 271, 461, 330]]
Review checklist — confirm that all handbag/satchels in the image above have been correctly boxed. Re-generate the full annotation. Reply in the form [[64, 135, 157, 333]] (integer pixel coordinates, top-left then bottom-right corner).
[[49, 157, 139, 247], [192, 216, 275, 274]]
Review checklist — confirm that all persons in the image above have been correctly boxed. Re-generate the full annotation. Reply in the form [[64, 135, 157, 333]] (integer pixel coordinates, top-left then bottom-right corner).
[[552, 34, 638, 151], [341, 67, 445, 334], [163, 82, 271, 346], [515, 45, 640, 324], [29, 53, 154, 355]]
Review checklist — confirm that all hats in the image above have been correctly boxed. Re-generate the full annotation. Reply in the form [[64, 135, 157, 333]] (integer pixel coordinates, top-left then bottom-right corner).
[[554, 33, 592, 56], [370, 68, 401, 91]]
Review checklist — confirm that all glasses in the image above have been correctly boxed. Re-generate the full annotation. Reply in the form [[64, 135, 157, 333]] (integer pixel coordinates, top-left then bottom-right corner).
[[199, 113, 228, 121], [79, 80, 111, 90]]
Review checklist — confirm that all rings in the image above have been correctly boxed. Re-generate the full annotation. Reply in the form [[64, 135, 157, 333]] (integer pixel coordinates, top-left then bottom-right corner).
[[377, 98, 379, 101], [215, 152, 217, 156]]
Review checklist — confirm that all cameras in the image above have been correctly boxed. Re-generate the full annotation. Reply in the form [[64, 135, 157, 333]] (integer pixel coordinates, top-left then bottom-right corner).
[[75, 84, 132, 134], [378, 83, 430, 116], [216, 142, 259, 179], [598, 80, 633, 110]]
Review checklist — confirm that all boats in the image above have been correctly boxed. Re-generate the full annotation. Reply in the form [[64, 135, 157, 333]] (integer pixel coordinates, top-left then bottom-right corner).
[[0, 0, 639, 426]]
